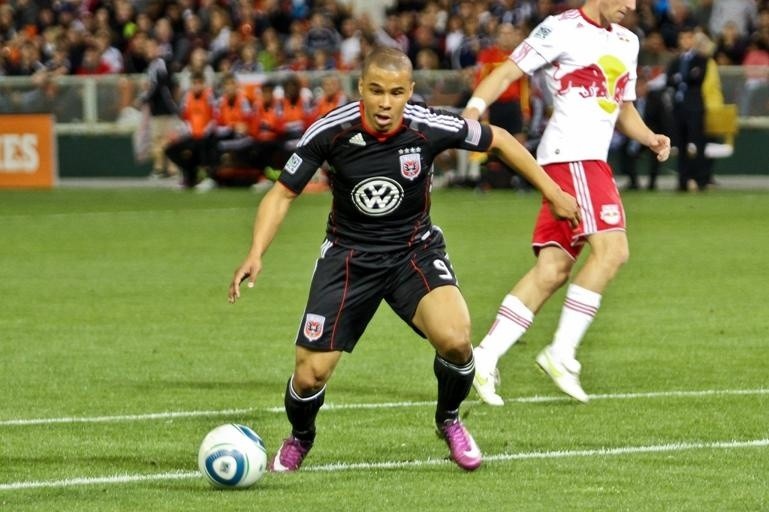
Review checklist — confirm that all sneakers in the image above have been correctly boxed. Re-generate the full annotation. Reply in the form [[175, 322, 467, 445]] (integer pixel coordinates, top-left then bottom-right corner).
[[269, 430, 316, 472], [534, 343, 588, 405], [433, 411, 483, 471], [472, 345, 505, 407]]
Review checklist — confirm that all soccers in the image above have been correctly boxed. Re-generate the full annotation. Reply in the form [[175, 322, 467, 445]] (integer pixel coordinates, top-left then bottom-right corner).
[[197, 423, 267, 488]]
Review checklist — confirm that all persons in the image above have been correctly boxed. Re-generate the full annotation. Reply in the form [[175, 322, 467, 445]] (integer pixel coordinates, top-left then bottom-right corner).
[[460, 2, 672, 409], [2, 0, 768, 194], [227, 45, 582, 474]]
